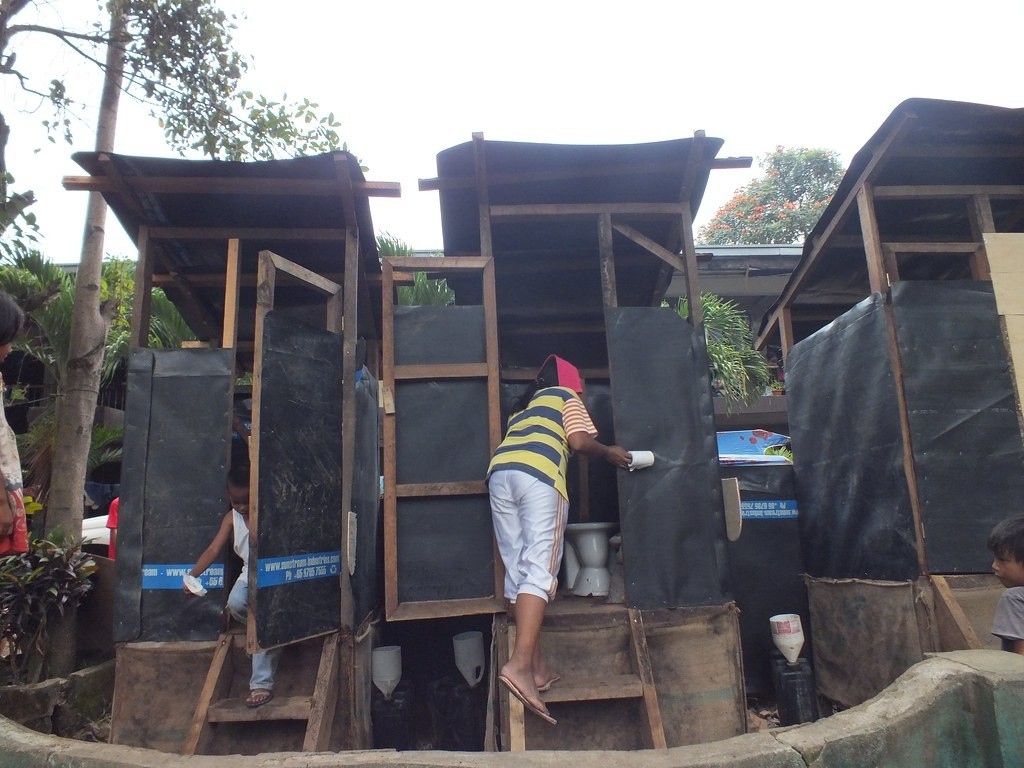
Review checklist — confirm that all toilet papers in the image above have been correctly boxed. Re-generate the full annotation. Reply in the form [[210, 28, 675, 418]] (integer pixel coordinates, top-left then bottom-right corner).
[[627, 450, 655, 472], [182, 572, 208, 597]]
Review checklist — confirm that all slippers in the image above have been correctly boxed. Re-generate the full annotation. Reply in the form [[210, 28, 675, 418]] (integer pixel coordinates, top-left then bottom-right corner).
[[246, 690, 272, 707], [536, 676, 561, 690], [498, 675, 558, 725]]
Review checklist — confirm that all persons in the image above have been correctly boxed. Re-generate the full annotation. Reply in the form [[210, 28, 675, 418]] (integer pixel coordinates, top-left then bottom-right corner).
[[106, 497, 120, 559], [0, 287, 30, 663], [485, 354, 632, 726], [182, 464, 282, 708], [988, 512, 1024, 655]]
